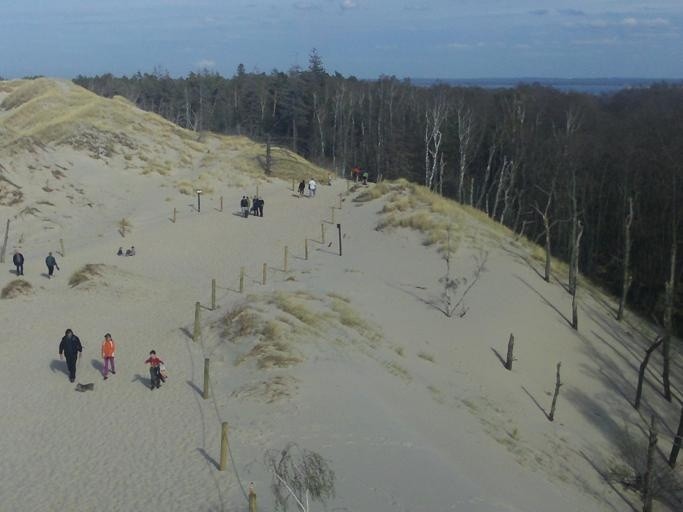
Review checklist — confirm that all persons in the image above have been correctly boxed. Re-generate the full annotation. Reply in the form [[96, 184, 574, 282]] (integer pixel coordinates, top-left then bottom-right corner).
[[240, 195, 265, 219], [115, 246, 135, 257], [327, 173, 333, 185], [13, 250, 24, 276], [45, 252, 59, 280], [144, 350, 164, 391], [353, 166, 368, 185], [101, 334, 116, 380], [298, 179, 316, 200], [59, 329, 82, 384]]
